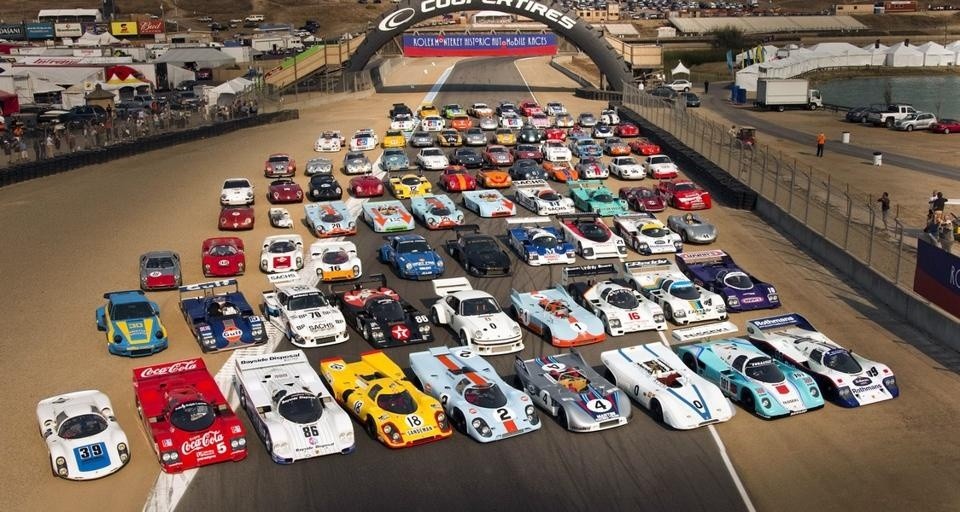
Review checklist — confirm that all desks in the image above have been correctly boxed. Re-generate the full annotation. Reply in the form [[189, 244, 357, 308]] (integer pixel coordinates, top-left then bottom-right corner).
[[913, 232, 960, 318]]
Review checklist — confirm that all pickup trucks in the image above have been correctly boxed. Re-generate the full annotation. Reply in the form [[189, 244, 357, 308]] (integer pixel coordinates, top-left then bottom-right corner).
[[870, 105, 924, 127]]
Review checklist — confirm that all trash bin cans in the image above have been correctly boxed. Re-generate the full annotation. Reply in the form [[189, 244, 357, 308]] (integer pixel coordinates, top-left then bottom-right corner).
[[841, 132, 850, 143], [873, 152, 882, 166], [731, 86, 746, 103]]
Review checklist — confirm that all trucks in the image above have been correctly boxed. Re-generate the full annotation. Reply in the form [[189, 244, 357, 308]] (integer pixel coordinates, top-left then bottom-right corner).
[[754, 76, 824, 111]]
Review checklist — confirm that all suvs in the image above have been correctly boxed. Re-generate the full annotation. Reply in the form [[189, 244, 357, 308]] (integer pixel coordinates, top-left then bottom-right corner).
[[897, 110, 938, 131]]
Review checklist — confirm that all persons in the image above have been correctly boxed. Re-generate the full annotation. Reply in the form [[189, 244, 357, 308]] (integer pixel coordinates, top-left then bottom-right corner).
[[728, 125, 737, 146], [815, 130, 827, 157], [877, 191, 890, 228], [923, 190, 953, 240], [704, 80, 709, 94], [4, 99, 259, 165]]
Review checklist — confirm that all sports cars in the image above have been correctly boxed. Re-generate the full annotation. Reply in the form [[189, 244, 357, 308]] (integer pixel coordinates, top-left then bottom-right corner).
[[671, 320, 827, 419], [131, 362, 248, 475], [598, 341, 735, 432], [407, 342, 542, 444], [37, 388, 130, 481], [511, 346, 633, 435], [233, 348, 355, 464], [317, 348, 453, 449], [744, 311, 900, 409]]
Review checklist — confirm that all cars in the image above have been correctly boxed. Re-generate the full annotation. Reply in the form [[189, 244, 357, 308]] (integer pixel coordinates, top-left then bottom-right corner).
[[1, 79, 202, 134], [930, 116, 960, 136], [561, 0, 788, 20], [403, 18, 457, 28], [196, 12, 321, 55], [652, 77, 700, 108]]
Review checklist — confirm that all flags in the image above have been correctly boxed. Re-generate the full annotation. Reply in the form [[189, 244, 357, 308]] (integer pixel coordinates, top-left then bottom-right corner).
[[726, 46, 767, 72]]
